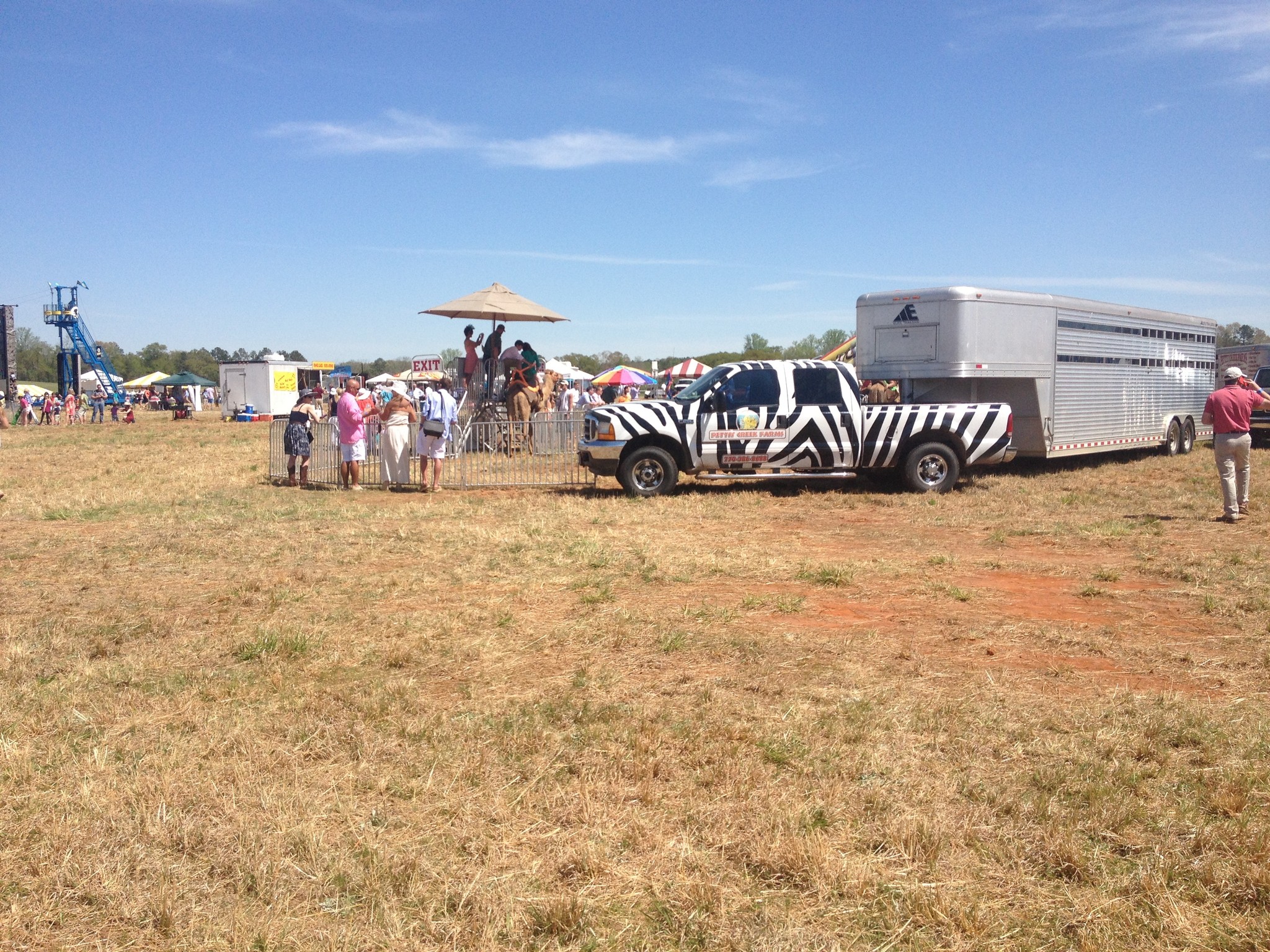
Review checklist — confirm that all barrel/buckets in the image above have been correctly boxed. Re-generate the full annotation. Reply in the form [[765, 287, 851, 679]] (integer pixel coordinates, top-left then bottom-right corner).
[[245, 405, 254, 414]]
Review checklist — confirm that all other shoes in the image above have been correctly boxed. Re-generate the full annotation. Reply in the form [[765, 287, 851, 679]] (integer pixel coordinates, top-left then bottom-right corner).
[[342, 487, 347, 491], [380, 485, 389, 490], [352, 485, 363, 490], [432, 485, 442, 493], [299, 482, 313, 486], [1216, 515, 1238, 524], [395, 486, 403, 492], [539, 389, 543, 398], [416, 484, 431, 492], [290, 483, 298, 487], [1238, 502, 1250, 514]]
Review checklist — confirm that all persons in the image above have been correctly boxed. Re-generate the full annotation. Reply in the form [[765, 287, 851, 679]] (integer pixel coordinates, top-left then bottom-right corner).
[[463, 324, 540, 399], [202, 386, 214, 411], [132, 387, 168, 410], [63, 297, 76, 320], [182, 388, 191, 403], [1201, 367, 1270, 525], [379, 381, 417, 492], [18, 384, 133, 427], [337, 379, 378, 491], [0, 393, 9, 499], [81, 387, 84, 394], [417, 378, 457, 493], [884, 380, 897, 391], [313, 382, 674, 451], [284, 388, 320, 487]]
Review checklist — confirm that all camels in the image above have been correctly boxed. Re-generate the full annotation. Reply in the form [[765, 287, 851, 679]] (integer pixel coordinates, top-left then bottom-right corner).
[[502, 368, 567, 458], [865, 379, 901, 405]]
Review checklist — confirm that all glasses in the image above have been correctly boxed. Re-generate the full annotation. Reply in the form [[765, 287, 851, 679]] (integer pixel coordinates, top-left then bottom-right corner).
[[305, 395, 313, 399]]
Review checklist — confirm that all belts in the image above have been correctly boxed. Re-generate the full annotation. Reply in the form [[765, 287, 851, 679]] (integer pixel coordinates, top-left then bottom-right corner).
[[1226, 431, 1247, 433], [289, 420, 304, 426]]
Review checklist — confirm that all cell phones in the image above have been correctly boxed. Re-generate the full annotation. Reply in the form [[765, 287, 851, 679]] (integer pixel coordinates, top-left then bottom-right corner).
[[366, 403, 371, 409], [1238, 377, 1249, 387]]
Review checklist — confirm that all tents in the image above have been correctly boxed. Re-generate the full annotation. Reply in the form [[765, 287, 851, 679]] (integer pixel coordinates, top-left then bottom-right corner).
[[366, 369, 452, 389], [151, 371, 220, 412], [656, 359, 713, 377], [81, 369, 123, 393], [16, 385, 54, 396], [117, 371, 171, 389]]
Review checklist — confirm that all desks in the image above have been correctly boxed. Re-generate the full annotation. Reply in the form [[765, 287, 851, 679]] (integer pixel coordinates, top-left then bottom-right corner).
[[169, 404, 193, 421]]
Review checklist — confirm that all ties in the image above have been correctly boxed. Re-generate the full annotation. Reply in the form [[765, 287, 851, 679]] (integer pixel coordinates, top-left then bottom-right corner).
[[589, 395, 592, 402]]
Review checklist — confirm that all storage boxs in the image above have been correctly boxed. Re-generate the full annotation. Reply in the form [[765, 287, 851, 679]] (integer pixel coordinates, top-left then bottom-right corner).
[[236, 413, 275, 423]]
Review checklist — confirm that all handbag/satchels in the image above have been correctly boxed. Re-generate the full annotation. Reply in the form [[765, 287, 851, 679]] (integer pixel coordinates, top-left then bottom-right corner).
[[423, 391, 444, 439], [307, 431, 314, 444]]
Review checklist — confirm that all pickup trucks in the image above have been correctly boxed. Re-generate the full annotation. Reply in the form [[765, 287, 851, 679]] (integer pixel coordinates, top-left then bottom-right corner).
[[1248, 365, 1270, 439], [579, 357, 1019, 495]]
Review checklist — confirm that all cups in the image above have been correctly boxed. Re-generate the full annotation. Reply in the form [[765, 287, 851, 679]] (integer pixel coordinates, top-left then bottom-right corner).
[[311, 405, 315, 410], [366, 404, 371, 409]]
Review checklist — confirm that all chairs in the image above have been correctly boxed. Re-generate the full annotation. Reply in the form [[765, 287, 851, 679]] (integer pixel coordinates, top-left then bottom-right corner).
[[748, 370, 839, 404], [148, 400, 159, 412]]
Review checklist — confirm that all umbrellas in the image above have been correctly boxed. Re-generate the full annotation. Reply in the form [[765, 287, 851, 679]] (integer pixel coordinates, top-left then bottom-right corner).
[[418, 282, 571, 398], [538, 358, 657, 395]]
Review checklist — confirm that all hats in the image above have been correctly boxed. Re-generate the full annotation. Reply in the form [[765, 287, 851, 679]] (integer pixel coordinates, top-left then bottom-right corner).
[[330, 388, 336, 395], [355, 388, 371, 400], [296, 389, 318, 404], [1224, 366, 1248, 381], [498, 325, 505, 332], [560, 380, 569, 386], [383, 381, 413, 403]]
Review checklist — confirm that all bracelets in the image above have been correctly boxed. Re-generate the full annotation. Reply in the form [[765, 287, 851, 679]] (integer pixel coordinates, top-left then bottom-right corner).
[[1257, 388, 1264, 393], [364, 412, 368, 416], [420, 423, 423, 426]]
